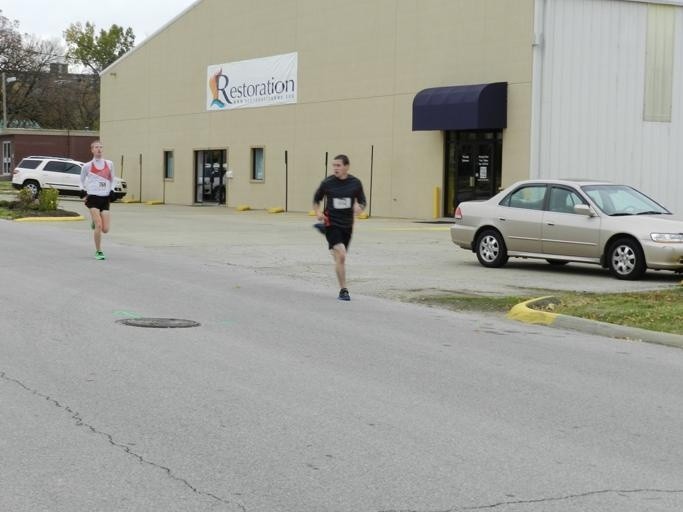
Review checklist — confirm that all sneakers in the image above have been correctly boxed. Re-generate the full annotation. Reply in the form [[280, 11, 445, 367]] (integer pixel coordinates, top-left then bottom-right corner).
[[95, 250, 106, 260], [338, 287, 351, 301], [313, 223, 325, 233], [91, 220, 95, 229]]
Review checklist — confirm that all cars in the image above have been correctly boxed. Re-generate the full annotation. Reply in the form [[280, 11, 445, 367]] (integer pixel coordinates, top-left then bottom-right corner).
[[204, 162, 227, 202], [450, 178, 683, 280], [11, 155, 127, 202]]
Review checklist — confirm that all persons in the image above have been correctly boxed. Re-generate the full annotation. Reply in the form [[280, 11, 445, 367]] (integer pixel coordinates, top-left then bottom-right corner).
[[311, 153, 367, 301], [78, 141, 115, 261]]
